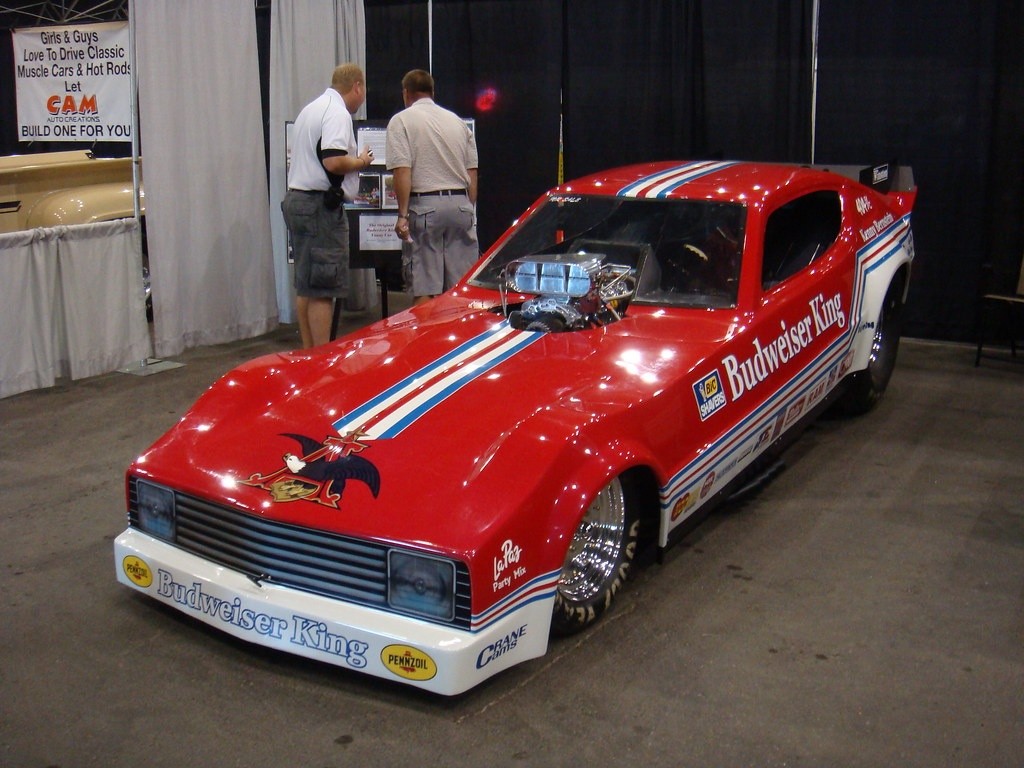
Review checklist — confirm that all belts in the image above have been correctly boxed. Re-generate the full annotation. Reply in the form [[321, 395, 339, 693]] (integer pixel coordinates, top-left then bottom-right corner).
[[408, 187, 469, 197]]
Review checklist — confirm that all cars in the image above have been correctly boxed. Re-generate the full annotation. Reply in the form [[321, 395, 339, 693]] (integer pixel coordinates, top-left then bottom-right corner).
[[106, 153, 913, 699]]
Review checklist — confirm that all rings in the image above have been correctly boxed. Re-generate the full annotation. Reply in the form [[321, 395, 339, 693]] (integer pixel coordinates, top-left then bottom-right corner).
[[398, 232, 401, 234]]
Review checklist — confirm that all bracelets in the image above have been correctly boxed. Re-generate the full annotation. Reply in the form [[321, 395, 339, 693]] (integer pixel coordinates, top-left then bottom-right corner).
[[357, 157, 365, 171]]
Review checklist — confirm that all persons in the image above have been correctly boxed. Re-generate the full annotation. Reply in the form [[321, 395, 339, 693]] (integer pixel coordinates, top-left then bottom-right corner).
[[280, 63, 375, 351], [386, 71, 478, 305]]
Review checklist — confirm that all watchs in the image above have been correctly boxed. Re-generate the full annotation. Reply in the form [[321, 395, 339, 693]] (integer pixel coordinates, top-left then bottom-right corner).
[[397, 212, 410, 219]]
